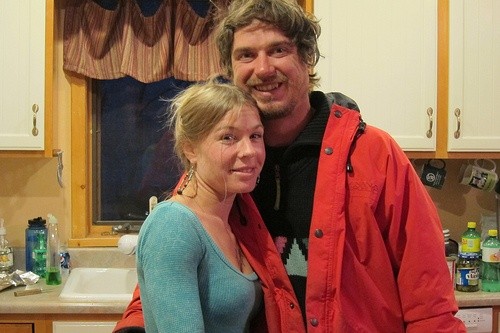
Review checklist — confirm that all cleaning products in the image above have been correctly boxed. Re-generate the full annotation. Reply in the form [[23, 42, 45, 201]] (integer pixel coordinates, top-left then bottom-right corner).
[[44, 216, 63, 284]]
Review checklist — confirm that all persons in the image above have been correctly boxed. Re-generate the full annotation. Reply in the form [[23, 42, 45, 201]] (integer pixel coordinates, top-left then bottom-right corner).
[[112, 0, 459, 333], [136, 81, 268, 333]]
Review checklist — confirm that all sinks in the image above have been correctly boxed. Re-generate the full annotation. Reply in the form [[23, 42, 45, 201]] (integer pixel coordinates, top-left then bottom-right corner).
[[59, 266, 139, 303]]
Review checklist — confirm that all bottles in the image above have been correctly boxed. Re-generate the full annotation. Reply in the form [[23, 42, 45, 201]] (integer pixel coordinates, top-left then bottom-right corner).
[[455, 222, 481, 293], [443, 229, 460, 288], [482, 230, 500, 293], [24, 214, 71, 285], [0, 218, 14, 279]]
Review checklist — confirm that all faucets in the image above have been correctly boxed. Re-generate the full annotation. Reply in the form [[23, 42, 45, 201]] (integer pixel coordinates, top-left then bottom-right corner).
[[116, 234, 140, 257]]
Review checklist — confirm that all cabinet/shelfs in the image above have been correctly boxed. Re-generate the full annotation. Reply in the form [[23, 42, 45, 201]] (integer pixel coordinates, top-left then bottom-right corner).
[[0, 0, 60, 159], [0, 302, 500, 333], [303, 0, 500, 161]]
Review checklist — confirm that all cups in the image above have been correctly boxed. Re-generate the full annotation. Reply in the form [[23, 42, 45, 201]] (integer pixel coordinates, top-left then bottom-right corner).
[[456, 159, 498, 193], [420, 159, 449, 190]]
